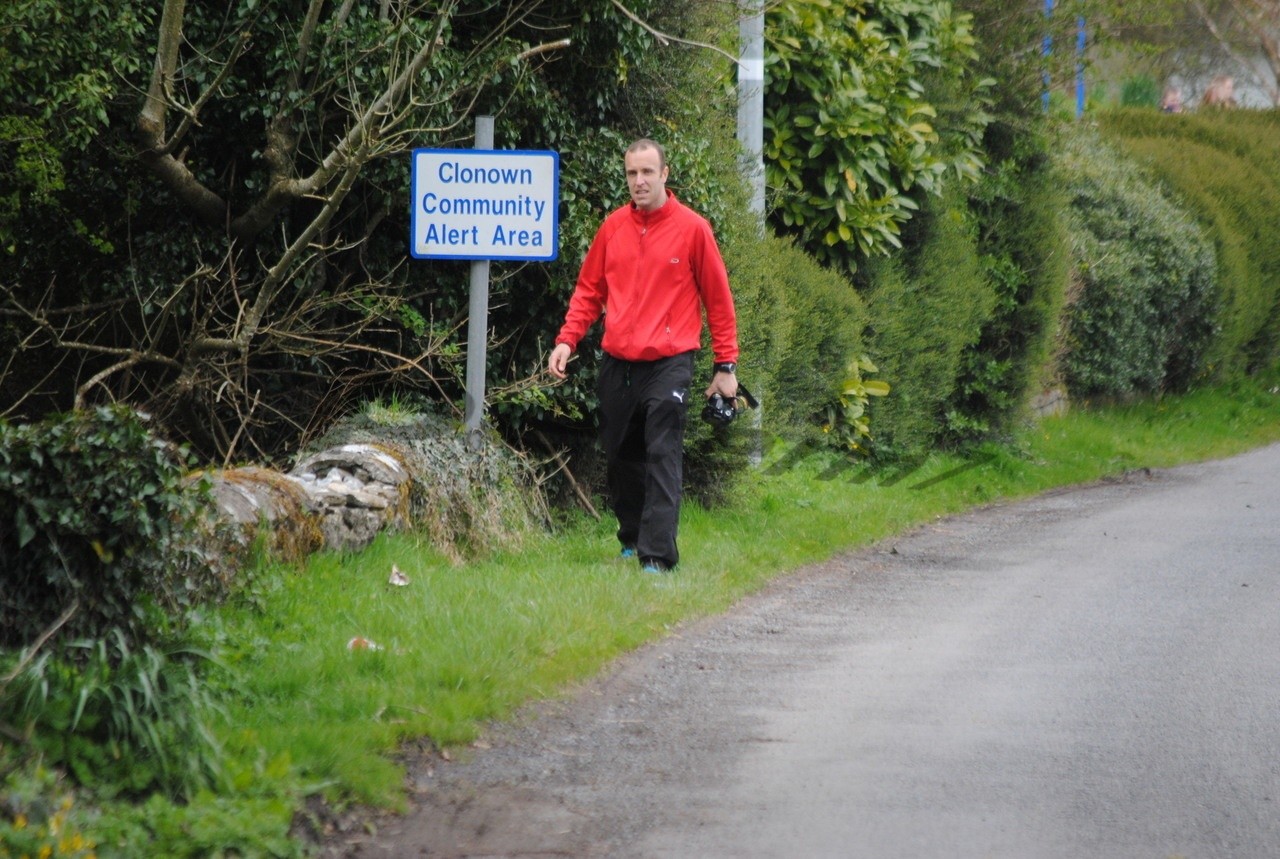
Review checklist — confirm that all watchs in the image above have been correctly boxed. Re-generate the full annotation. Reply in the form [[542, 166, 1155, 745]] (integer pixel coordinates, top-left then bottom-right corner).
[[716, 362, 736, 373]]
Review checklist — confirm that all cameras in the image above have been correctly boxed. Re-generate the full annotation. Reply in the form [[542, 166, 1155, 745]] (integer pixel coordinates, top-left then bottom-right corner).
[[700, 381, 760, 430]]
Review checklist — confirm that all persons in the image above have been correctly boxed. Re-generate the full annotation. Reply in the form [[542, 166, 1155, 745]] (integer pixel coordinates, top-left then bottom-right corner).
[[548, 139, 739, 574]]
